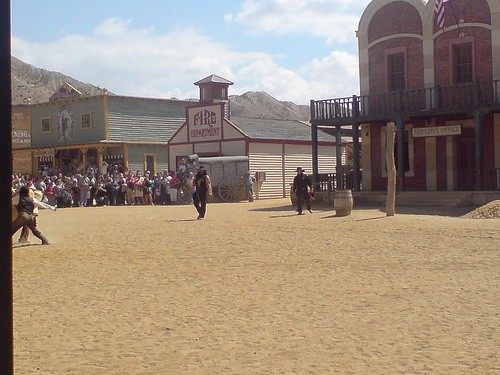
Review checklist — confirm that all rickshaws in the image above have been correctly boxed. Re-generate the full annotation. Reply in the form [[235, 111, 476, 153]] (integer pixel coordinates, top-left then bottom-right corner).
[[177, 153, 250, 203]]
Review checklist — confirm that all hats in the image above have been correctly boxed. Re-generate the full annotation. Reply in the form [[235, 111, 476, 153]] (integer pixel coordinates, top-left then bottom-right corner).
[[294, 167, 305, 172], [197, 165, 206, 171]]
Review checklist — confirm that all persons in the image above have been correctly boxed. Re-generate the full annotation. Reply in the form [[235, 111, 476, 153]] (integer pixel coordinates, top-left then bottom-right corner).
[[192, 166, 212, 220], [11, 186, 56, 245], [11, 161, 197, 208], [293, 167, 313, 214]]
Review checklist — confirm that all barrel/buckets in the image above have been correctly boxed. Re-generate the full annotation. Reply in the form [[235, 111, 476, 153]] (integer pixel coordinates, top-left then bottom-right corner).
[[335, 190, 353, 217]]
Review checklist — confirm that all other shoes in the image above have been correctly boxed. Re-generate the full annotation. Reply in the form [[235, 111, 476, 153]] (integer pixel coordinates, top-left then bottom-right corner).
[[148, 201, 154, 206], [197, 215, 205, 220], [308, 209, 312, 213], [41, 241, 50, 245], [297, 208, 302, 214]]
